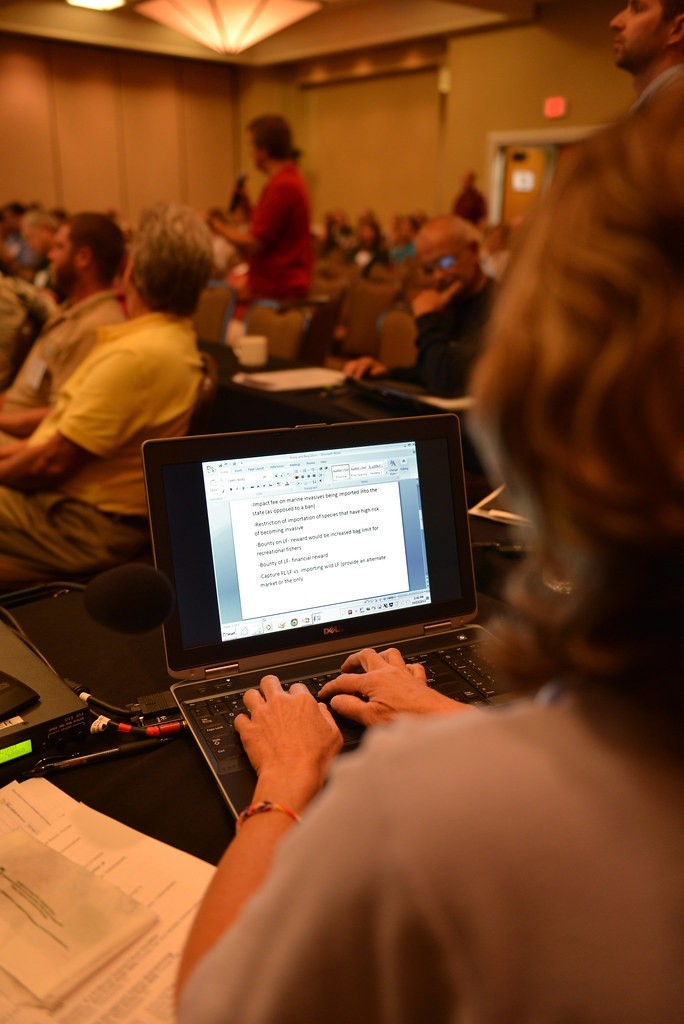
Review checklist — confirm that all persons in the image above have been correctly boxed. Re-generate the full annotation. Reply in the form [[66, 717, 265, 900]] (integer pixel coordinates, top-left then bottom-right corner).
[[450, 171, 487, 224], [227, 176, 254, 215], [171, 81, 684, 1024], [605, 0, 684, 106], [0, 204, 532, 605], [203, 114, 316, 312]]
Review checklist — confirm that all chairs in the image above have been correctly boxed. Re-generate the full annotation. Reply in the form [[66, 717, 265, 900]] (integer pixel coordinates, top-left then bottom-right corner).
[[340, 282, 402, 355], [245, 301, 310, 362], [193, 281, 237, 346]]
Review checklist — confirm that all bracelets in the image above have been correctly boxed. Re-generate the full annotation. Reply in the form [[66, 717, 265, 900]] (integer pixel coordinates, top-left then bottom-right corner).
[[236, 801, 302, 831]]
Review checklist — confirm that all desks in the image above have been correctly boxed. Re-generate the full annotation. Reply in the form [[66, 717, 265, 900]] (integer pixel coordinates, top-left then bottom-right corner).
[[224, 377, 420, 422]]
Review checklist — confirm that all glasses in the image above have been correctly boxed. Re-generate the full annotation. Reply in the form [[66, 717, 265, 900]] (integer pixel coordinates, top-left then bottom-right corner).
[[421, 256, 456, 274]]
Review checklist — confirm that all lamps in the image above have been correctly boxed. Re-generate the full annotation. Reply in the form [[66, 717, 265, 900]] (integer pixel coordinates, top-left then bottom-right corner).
[[133, 0, 322, 56]]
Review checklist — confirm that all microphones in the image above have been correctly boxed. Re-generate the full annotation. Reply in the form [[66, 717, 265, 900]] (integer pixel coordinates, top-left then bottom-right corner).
[[0, 563, 175, 635], [229, 173, 248, 212]]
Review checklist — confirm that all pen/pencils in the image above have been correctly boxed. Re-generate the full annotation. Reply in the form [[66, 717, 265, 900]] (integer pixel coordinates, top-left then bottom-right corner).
[[20, 736, 175, 778]]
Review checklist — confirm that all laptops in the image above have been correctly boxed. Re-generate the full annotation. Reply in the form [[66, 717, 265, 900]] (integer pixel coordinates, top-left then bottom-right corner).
[[139, 413, 520, 823]]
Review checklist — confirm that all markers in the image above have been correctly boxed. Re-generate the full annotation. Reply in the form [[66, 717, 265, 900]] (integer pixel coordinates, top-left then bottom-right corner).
[[319, 388, 348, 398]]
